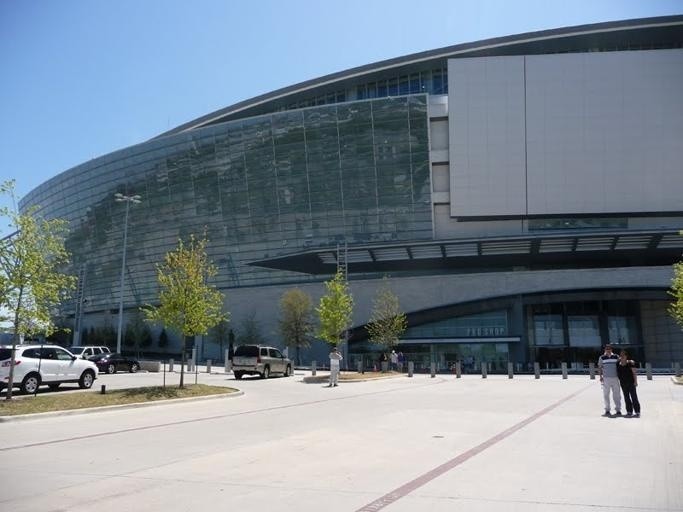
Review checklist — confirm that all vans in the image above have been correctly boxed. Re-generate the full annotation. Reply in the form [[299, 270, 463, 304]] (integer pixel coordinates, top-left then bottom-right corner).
[[68, 344, 111, 359]]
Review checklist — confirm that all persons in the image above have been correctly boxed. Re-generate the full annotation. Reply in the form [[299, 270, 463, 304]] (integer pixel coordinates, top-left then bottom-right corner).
[[616, 351, 640, 418], [596, 343, 634, 418], [380, 350, 404, 373], [329, 348, 343, 386]]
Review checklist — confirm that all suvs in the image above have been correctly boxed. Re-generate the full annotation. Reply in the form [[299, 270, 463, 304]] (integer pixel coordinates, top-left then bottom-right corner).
[[230, 344, 291, 379], [0, 344, 98, 394]]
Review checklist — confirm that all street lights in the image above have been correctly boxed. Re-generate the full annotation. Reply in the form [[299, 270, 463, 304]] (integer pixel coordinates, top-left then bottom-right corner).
[[112, 192, 141, 353]]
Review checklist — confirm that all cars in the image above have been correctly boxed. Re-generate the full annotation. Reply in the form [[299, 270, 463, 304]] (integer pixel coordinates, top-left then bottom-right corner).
[[87, 352, 138, 374]]
